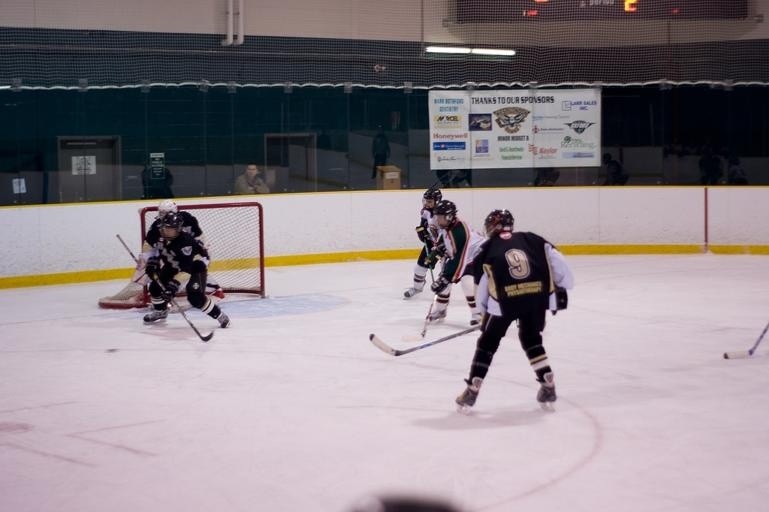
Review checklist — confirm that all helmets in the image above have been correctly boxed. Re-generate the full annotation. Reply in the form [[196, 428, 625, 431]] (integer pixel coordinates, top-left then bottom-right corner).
[[424, 188, 443, 205], [158, 200, 178, 219], [484, 208, 515, 237], [432, 200, 457, 221], [156, 212, 184, 229]]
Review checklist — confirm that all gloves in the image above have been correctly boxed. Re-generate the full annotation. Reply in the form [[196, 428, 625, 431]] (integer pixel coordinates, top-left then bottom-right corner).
[[431, 274, 450, 294], [425, 249, 443, 268], [416, 226, 429, 241], [160, 289, 176, 302], [146, 256, 162, 277]]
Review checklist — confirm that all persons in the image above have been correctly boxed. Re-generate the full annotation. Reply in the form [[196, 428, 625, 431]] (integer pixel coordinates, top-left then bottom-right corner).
[[403, 190, 451, 301], [143, 211, 229, 328], [138, 200, 203, 314], [234, 162, 271, 194], [142, 155, 176, 199], [455, 208, 578, 408], [423, 199, 487, 325]]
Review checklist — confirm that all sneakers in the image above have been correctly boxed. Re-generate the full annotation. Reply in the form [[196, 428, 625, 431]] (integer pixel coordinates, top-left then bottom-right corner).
[[404, 288, 423, 297], [144, 309, 167, 321], [470, 310, 482, 325], [217, 311, 229, 328], [426, 306, 446, 320]]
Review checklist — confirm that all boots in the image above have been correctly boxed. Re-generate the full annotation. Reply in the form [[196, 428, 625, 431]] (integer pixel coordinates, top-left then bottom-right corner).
[[456, 377, 483, 406], [536, 371, 557, 401]]
